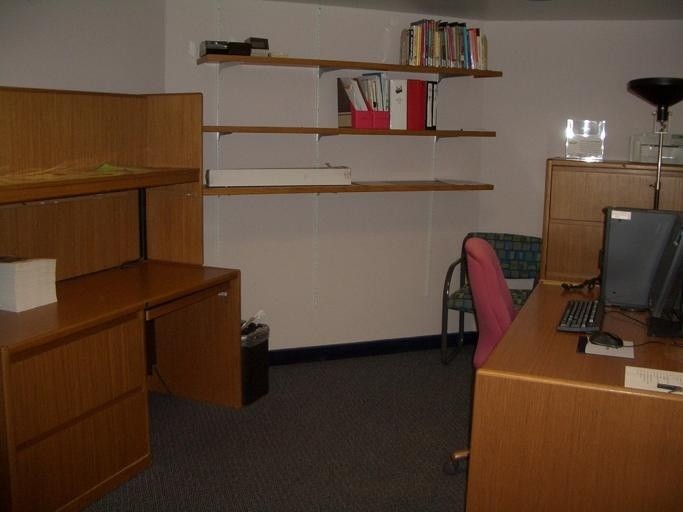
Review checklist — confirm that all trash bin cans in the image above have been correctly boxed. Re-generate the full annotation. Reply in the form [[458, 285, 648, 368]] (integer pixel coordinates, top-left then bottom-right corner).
[[241, 321, 269, 405]]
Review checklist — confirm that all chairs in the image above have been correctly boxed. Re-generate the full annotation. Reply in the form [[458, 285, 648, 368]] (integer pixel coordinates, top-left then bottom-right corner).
[[441, 232, 542, 477]]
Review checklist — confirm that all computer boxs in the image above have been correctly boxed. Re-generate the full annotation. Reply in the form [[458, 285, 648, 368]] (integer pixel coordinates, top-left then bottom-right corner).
[[601, 206, 683, 310]]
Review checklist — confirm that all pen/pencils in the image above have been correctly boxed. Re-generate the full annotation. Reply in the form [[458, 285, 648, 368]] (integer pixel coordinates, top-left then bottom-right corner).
[[657, 383, 683, 393]]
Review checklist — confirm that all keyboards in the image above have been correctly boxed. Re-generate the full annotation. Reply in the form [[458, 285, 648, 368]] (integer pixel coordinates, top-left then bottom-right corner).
[[556, 299, 603, 331]]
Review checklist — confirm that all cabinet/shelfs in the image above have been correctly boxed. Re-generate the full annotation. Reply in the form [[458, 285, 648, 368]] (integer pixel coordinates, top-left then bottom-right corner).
[[0, 86, 204, 264], [542, 154, 683, 293], [2, 313, 153, 511], [197, 54, 503, 196]]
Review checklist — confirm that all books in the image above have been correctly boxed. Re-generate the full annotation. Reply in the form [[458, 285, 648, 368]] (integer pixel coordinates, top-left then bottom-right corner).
[[622, 362, 683, 400], [334, 17, 488, 132], [0, 256, 59, 314]]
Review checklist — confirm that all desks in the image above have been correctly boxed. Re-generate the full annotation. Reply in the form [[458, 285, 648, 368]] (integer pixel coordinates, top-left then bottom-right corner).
[[464, 281, 683, 509], [0, 258, 241, 415]]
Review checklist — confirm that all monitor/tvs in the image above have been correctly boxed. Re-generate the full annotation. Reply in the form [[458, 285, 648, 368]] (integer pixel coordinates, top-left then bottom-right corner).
[[649, 212, 683, 338]]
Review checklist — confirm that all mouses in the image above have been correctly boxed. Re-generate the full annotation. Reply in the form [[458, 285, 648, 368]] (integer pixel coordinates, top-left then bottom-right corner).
[[586, 331, 623, 347]]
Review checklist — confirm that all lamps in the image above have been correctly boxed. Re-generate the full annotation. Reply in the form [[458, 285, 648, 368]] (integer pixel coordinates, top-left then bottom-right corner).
[[626, 77, 683, 212]]
